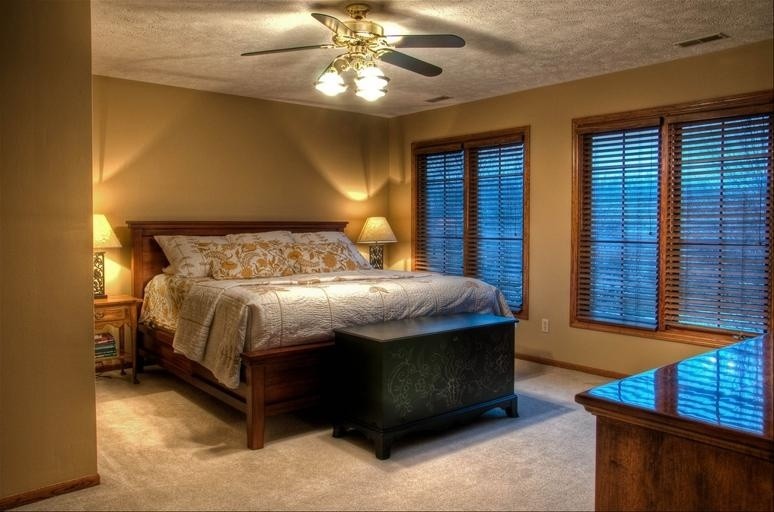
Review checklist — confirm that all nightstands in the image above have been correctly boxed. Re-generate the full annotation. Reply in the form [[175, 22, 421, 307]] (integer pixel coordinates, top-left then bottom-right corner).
[[94, 295, 144, 384]]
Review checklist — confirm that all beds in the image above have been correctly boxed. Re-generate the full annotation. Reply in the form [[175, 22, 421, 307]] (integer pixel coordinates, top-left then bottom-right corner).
[[125, 221, 494, 451]]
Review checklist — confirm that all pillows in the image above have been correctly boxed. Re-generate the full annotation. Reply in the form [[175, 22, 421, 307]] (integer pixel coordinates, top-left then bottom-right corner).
[[152, 230, 373, 281]]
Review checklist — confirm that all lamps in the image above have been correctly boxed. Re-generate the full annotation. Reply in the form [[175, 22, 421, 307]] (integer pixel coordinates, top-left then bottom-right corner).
[[314, 2, 391, 101], [92, 214, 123, 299], [356, 217, 398, 270]]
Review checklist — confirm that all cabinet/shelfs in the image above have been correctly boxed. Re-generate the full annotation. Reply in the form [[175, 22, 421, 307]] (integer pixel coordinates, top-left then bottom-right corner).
[[333, 313, 518, 460]]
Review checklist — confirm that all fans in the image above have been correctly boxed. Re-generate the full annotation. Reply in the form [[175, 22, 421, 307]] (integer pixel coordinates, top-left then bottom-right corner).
[[242, 3, 465, 77]]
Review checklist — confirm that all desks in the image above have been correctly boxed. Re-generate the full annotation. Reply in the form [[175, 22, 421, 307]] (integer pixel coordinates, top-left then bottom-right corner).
[[574, 333, 774, 512]]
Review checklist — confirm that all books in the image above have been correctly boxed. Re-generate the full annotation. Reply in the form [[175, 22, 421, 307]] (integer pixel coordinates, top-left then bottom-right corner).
[[95, 332, 117, 358]]
